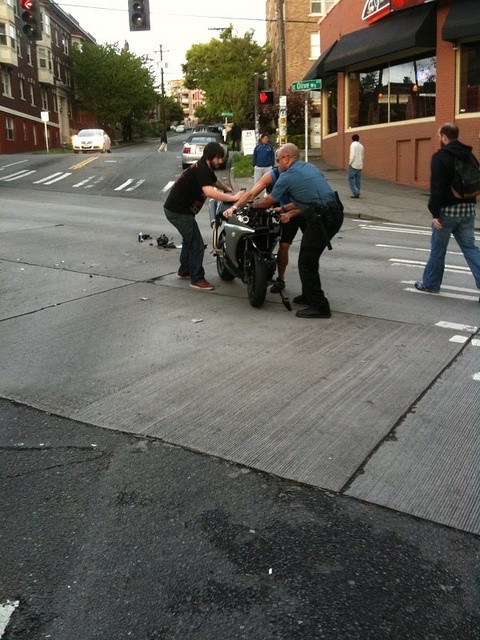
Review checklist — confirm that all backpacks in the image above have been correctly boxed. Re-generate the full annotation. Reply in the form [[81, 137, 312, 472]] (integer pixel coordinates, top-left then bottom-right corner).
[[443, 147, 480, 199]]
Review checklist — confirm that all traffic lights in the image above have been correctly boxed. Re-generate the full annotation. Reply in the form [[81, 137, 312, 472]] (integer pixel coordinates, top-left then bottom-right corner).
[[20, 1, 42, 41], [127, 0, 151, 31], [258, 90, 274, 106]]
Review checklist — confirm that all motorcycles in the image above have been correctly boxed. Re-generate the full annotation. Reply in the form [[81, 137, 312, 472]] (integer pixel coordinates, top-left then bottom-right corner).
[[209, 188, 293, 312]]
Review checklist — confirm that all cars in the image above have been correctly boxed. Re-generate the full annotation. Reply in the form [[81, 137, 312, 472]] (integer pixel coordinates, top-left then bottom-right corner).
[[71, 128, 112, 154], [181, 132, 230, 170]]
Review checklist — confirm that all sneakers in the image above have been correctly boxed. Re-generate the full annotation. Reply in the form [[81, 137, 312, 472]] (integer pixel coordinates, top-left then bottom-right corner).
[[415, 282, 439, 292], [178, 273, 189, 278], [271, 280, 286, 293], [190, 277, 214, 290]]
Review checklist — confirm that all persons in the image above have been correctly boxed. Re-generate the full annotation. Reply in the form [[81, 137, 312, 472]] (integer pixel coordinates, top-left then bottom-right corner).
[[346, 134, 364, 198], [251, 143, 344, 319], [253, 134, 275, 195], [222, 148, 309, 293], [230, 122, 242, 152], [157, 127, 167, 153], [163, 142, 245, 290], [414, 123, 480, 304]]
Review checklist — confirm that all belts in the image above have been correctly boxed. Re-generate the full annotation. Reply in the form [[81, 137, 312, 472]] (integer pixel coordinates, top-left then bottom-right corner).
[[263, 165, 270, 166]]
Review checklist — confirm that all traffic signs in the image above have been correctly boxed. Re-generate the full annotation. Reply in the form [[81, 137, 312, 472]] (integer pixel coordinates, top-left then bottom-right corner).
[[291, 79, 322, 92]]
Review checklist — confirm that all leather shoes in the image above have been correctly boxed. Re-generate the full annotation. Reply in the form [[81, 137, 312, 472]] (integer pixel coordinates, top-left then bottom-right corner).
[[294, 295, 312, 306], [297, 307, 330, 318]]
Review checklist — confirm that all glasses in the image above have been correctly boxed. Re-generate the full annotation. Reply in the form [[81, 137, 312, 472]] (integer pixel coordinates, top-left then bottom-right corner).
[[276, 156, 284, 163]]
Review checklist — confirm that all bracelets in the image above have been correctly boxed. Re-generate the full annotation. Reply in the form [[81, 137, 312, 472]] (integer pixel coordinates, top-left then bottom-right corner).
[[231, 205, 239, 211], [281, 206, 289, 213]]
